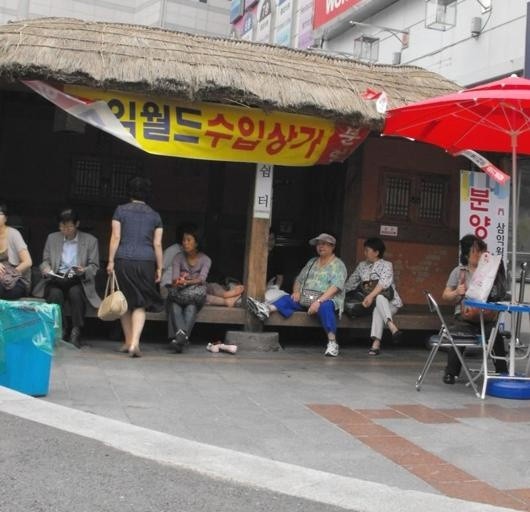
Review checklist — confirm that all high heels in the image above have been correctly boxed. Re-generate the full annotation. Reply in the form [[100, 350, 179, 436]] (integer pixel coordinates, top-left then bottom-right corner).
[[112, 341, 131, 353]]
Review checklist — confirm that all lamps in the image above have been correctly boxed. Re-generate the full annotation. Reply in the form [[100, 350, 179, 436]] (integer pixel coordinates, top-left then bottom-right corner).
[[422, 0, 494, 38], [345, 16, 409, 64]]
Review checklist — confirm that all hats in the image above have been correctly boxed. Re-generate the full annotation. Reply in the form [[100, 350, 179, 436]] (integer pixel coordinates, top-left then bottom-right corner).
[[306, 231, 337, 247]]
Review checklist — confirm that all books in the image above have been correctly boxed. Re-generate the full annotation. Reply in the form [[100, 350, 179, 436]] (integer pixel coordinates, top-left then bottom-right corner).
[[47, 265, 79, 280]]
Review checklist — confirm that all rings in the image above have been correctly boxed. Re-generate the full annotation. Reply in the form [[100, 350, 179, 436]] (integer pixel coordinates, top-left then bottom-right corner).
[[309, 309, 310, 311]]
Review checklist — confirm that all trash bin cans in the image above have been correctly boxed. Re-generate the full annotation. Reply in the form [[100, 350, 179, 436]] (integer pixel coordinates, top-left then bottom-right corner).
[[0, 299, 61, 396]]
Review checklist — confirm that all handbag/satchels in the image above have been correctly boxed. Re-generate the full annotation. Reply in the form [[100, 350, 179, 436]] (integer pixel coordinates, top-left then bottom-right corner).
[[457, 298, 501, 326], [166, 279, 209, 306], [96, 290, 128, 322], [359, 272, 394, 302], [0, 260, 24, 291], [297, 287, 324, 309]]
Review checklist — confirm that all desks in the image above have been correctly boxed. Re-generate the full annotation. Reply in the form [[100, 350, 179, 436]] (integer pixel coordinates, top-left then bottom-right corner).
[[460, 297, 530, 399]]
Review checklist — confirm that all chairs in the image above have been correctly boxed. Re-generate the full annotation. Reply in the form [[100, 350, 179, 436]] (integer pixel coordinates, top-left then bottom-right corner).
[[414, 289, 481, 394]]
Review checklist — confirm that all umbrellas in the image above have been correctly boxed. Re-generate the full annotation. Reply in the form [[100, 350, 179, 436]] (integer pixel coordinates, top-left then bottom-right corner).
[[377, 73, 529, 401]]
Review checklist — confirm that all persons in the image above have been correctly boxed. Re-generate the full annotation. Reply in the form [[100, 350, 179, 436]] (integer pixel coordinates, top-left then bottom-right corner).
[[107, 176, 165, 357], [167, 226, 212, 349], [0, 203, 34, 299], [441, 233, 508, 385], [244, 232, 349, 357], [160, 221, 244, 309], [34, 208, 99, 344], [346, 236, 403, 355]]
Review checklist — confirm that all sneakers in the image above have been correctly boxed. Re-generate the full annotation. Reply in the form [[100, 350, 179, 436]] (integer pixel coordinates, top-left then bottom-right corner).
[[324, 340, 340, 358], [218, 343, 239, 356], [126, 343, 143, 358], [245, 296, 271, 322], [69, 330, 81, 350], [205, 343, 220, 353], [175, 333, 190, 346], [391, 329, 404, 341], [443, 372, 456, 385], [367, 347, 380, 356]]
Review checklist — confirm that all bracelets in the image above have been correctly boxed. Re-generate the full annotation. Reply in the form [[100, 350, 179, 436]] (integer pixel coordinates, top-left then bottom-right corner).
[[317, 299, 322, 304]]
